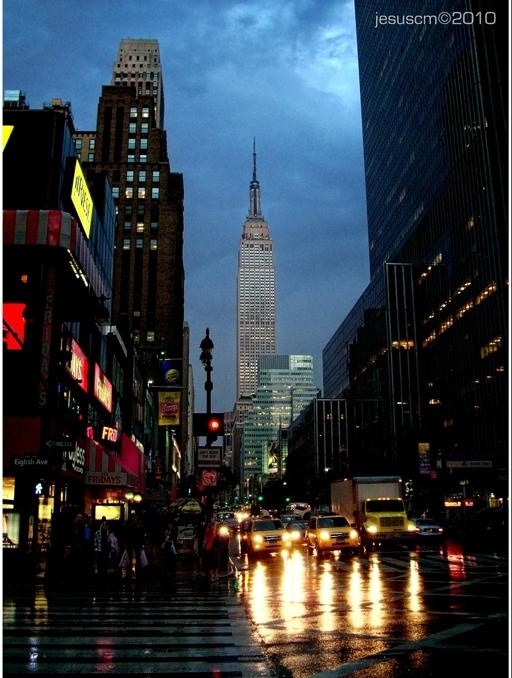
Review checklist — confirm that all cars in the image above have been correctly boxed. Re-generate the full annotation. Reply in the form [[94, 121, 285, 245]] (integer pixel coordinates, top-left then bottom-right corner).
[[411, 518, 446, 540], [213, 501, 362, 560]]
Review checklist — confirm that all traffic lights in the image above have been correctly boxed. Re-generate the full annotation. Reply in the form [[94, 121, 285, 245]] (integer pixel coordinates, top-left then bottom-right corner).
[[32, 480, 44, 495], [208, 413, 223, 433]]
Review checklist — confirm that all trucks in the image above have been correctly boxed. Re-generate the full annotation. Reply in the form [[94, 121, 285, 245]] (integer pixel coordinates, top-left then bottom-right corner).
[[331, 475, 409, 553]]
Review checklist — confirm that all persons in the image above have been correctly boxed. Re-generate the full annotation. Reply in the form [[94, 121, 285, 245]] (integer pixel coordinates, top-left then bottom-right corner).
[[62, 501, 230, 595]]
